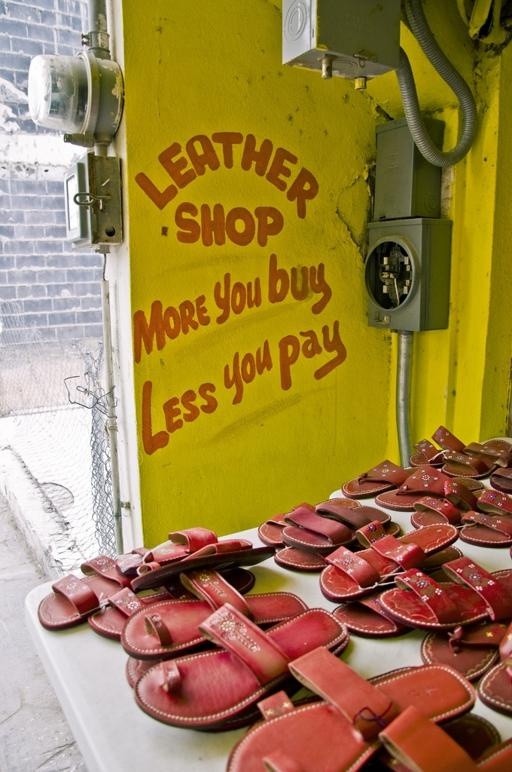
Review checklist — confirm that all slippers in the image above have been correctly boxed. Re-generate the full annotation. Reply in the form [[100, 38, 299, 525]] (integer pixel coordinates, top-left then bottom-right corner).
[[405, 479, 512, 544], [222, 651, 512, 772], [40, 518, 272, 627], [409, 427, 510, 492], [256, 497, 398, 567], [318, 523, 512, 718], [345, 461, 481, 507], [122, 568, 348, 735]]
